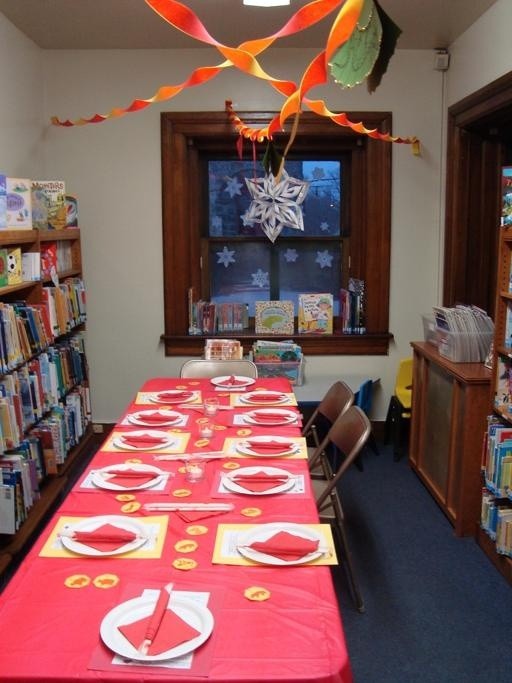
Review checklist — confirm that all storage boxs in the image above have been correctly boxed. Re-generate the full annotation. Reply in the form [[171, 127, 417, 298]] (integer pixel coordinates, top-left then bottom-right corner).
[[436, 326, 494, 363], [244, 347, 307, 387], [422, 311, 443, 344]]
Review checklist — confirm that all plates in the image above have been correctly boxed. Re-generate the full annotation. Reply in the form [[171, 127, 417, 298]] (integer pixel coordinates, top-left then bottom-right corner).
[[223, 465, 296, 495], [99, 593, 215, 660], [93, 462, 164, 491], [240, 520, 326, 567], [63, 515, 151, 554], [111, 373, 301, 458]]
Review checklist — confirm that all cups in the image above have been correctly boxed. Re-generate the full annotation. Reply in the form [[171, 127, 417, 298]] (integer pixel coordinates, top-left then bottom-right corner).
[[184, 455, 205, 481]]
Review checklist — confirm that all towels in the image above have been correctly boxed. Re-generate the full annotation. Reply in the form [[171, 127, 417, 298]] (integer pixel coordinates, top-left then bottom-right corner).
[[74, 386, 193, 556], [218, 374, 319, 561], [145, 588, 173, 643], [117, 588, 200, 655]]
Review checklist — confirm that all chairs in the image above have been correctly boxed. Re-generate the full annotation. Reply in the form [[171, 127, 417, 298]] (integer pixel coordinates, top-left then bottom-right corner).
[[179, 358, 258, 381], [384, 356, 416, 459], [305, 379, 359, 477], [304, 402, 375, 620]]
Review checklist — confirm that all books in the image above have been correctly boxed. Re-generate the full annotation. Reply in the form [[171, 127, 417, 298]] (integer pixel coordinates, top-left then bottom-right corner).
[[0, 170, 92, 540], [431, 168, 511, 560], [185, 278, 366, 384]]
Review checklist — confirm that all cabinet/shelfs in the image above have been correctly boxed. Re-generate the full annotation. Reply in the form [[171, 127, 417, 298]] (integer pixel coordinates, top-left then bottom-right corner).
[[410, 339, 494, 540], [451, 162, 512, 588], [1, 224, 91, 565]]
